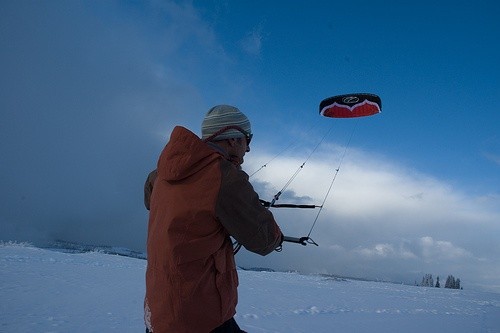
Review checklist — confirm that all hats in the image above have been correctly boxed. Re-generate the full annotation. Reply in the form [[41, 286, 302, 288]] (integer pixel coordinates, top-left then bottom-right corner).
[[201, 105, 251, 141]]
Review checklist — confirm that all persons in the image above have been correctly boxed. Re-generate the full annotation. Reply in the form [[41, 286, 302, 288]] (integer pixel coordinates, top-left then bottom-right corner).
[[144, 105, 283, 333]]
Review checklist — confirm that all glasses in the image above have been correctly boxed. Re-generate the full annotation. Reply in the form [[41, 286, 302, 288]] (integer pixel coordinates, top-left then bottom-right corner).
[[246, 132, 253, 145]]
